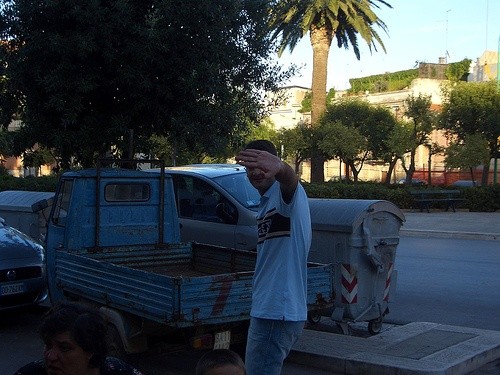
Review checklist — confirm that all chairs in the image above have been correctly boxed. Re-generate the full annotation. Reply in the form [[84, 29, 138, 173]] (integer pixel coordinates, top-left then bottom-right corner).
[[173, 177, 192, 217], [197, 182, 217, 216]]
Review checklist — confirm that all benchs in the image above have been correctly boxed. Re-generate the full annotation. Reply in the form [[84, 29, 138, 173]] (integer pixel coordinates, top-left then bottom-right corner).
[[416, 198, 467, 213]]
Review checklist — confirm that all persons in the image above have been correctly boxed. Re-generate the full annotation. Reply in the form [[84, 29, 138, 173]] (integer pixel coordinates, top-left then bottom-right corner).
[[196, 349, 247, 375], [14, 305, 144, 375], [235, 139, 312, 375]]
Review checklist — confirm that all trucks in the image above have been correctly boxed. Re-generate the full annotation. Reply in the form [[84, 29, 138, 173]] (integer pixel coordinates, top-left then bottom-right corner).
[[31, 156, 341, 368]]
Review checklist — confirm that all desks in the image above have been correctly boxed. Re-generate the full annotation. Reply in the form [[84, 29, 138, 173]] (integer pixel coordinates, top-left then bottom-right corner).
[[410, 190, 460, 213]]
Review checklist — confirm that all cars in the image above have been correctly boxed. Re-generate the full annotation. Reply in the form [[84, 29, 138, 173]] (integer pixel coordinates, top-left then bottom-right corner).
[[454, 180, 480, 188], [397, 177, 428, 186], [0, 215, 49, 315], [143, 163, 262, 251]]
